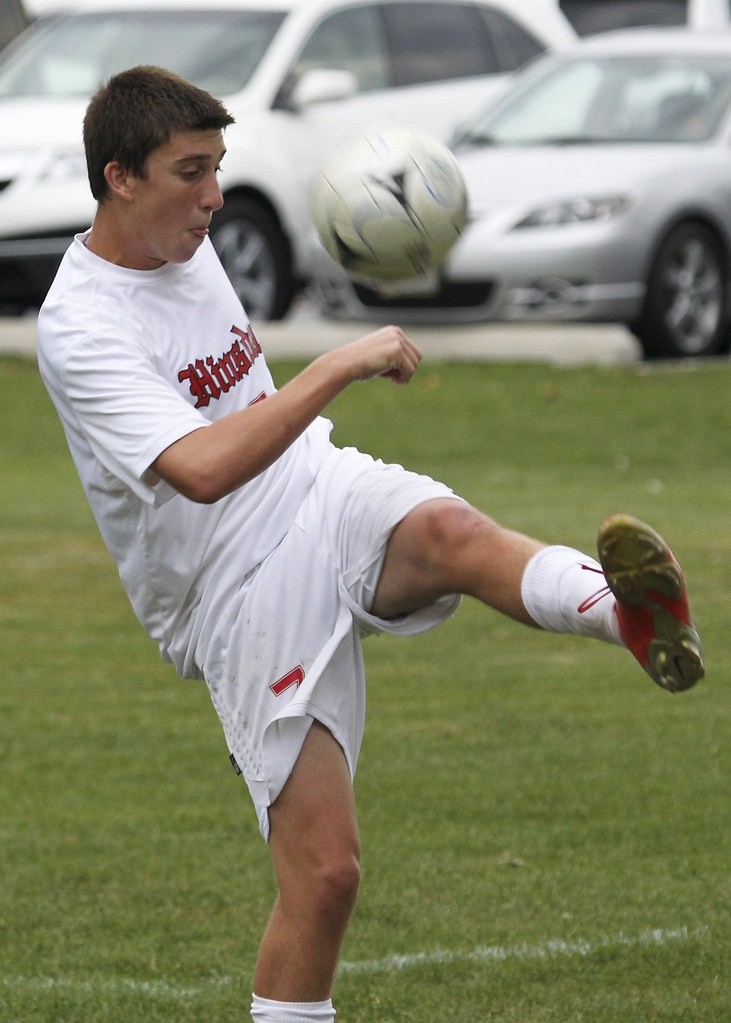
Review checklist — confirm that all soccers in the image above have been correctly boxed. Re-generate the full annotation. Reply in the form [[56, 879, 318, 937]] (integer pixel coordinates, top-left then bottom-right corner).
[[315, 126, 476, 281]]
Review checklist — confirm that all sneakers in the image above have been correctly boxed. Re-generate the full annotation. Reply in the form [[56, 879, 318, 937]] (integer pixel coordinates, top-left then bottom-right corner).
[[598, 514, 706, 692]]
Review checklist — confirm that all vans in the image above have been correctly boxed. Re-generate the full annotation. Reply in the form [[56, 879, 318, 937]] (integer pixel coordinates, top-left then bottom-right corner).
[[0, 0, 585, 325]]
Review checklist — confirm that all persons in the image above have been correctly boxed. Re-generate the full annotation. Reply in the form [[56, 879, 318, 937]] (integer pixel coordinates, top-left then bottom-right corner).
[[34, 64, 706, 1023]]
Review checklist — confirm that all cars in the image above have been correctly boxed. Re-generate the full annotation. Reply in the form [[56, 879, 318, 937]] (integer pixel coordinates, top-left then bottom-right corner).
[[313, 24, 729, 363]]
